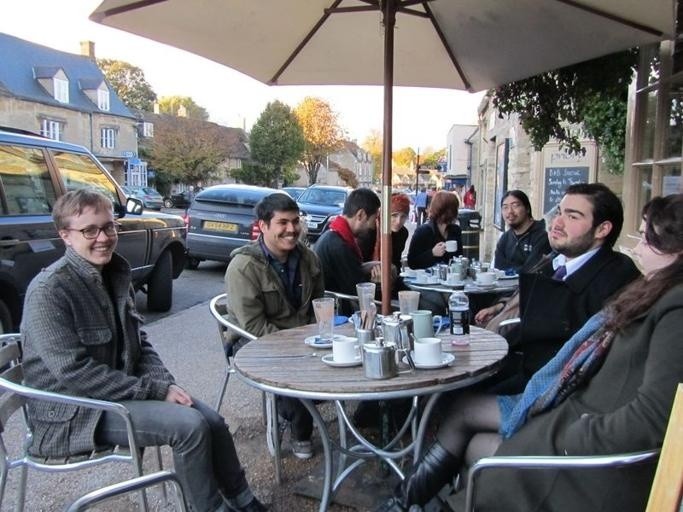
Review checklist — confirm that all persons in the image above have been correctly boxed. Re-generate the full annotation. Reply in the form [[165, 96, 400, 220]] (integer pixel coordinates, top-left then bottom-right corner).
[[407, 192, 463, 314], [429, 186, 438, 197], [475, 205, 568, 334], [474, 183, 645, 396], [456, 183, 464, 202], [313, 188, 382, 317], [20, 189, 270, 512], [409, 203, 417, 225], [414, 188, 429, 227], [193, 181, 204, 197], [494, 190, 558, 279], [356, 191, 447, 319], [376, 192, 683, 511], [464, 184, 476, 210], [184, 186, 196, 215], [224, 193, 326, 460]]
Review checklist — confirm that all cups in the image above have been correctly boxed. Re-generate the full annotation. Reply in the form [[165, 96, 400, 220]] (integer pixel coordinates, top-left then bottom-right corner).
[[416, 273, 429, 282], [410, 310, 443, 339], [476, 273, 496, 283], [414, 338, 443, 365], [443, 241, 458, 253], [312, 298, 336, 343], [355, 282, 378, 311], [332, 335, 362, 361], [446, 273, 461, 284], [398, 291, 420, 315]]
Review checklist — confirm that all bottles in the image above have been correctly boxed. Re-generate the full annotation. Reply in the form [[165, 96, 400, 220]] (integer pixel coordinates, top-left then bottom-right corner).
[[447, 284, 470, 346]]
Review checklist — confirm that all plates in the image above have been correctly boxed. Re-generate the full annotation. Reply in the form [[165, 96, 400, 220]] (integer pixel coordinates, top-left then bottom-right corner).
[[498, 274, 520, 279], [401, 352, 456, 369], [473, 281, 499, 287], [399, 272, 423, 278], [321, 355, 362, 367], [303, 334, 346, 348], [439, 281, 466, 287], [411, 278, 440, 285]]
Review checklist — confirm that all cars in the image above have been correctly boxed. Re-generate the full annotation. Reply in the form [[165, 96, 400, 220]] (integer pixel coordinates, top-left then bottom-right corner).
[[183, 183, 306, 269], [283, 187, 305, 202], [132, 188, 162, 210], [163, 190, 189, 208]]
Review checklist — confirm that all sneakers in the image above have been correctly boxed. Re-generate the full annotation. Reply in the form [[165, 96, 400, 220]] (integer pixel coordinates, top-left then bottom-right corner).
[[242, 496, 269, 512], [290, 437, 314, 458]]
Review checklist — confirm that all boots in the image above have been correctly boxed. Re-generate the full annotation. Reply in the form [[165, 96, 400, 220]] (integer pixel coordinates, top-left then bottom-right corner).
[[374, 434, 461, 511]]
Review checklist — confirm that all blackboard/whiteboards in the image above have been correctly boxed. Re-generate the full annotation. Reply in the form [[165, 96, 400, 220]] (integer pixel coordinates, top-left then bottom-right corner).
[[543, 166, 589, 216], [492, 137, 510, 233]]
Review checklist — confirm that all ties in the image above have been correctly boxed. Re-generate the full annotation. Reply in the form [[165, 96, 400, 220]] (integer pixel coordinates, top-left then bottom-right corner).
[[549, 266, 566, 280]]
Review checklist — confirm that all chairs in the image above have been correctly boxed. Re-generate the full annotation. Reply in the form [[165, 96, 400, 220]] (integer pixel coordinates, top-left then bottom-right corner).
[[467, 384, 683, 512], [67, 466, 189, 512], [208, 291, 360, 489], [0, 332, 166, 512]]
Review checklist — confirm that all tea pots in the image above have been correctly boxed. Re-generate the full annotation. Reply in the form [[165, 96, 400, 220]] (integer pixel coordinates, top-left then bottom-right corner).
[[375, 311, 415, 353], [358, 337, 416, 381]]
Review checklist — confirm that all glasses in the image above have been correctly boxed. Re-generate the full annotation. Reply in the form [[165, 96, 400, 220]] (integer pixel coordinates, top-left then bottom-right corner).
[[66, 221, 121, 240]]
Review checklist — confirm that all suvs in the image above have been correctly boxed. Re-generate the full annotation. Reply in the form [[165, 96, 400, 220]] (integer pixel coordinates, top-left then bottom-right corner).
[[297, 185, 354, 236], [0, 127, 186, 333]]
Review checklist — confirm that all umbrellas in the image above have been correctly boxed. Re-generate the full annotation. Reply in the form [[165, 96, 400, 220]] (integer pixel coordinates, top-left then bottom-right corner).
[[86, 0, 679, 313]]
